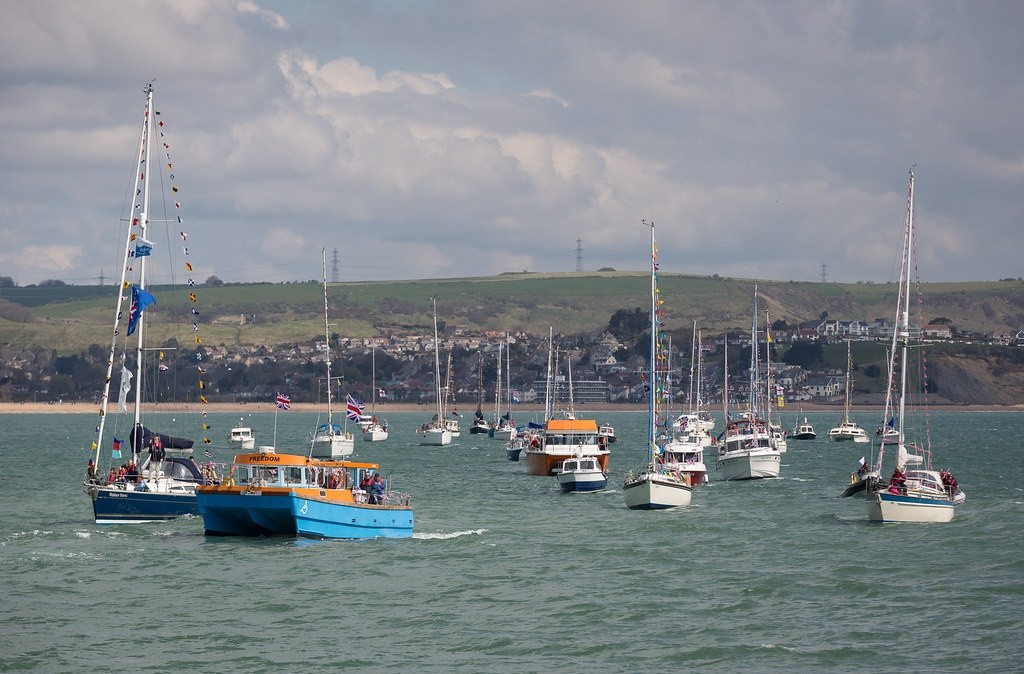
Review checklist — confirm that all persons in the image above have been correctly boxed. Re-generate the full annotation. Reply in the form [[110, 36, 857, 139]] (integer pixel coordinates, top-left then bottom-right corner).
[[742, 424, 753, 435], [939, 468, 958, 498], [415, 421, 429, 435], [147, 435, 165, 483], [858, 461, 870, 481], [361, 414, 389, 433], [758, 423, 766, 433], [889, 466, 908, 496], [87, 459, 102, 485], [728, 424, 740, 435], [110, 457, 139, 483], [361, 471, 385, 504], [711, 437, 716, 444], [190, 454, 219, 486], [322, 468, 344, 489], [876, 427, 883, 434]]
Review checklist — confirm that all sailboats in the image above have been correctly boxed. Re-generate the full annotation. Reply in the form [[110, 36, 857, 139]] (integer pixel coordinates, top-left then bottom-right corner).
[[839, 161, 966, 523], [621, 217, 787, 511], [826, 339, 870, 443], [875, 345, 905, 446], [414, 297, 617, 478], [362, 338, 388, 441], [307, 245, 355, 458], [82, 79, 213, 525]]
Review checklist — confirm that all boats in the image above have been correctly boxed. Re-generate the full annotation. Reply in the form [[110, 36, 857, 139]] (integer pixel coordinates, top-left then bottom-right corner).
[[227, 417, 255, 450], [194, 401, 414, 541], [355, 415, 373, 430], [792, 407, 817, 440], [556, 442, 608, 491]]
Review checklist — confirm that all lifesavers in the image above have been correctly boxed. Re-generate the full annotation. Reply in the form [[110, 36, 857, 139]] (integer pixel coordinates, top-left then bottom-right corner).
[[719, 446, 727, 456], [224, 477, 235, 486]]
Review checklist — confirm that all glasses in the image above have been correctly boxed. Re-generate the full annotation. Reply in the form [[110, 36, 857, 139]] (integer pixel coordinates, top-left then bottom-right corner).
[[366, 475, 370, 476]]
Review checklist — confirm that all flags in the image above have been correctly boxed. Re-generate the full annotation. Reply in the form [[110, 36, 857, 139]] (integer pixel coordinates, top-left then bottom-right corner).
[[443, 360, 458, 414], [346, 395, 366, 424], [651, 242, 687, 465], [892, 184, 934, 469], [88, 102, 216, 460], [690, 299, 853, 422], [512, 394, 519, 403], [475, 356, 574, 419], [276, 390, 291, 411]]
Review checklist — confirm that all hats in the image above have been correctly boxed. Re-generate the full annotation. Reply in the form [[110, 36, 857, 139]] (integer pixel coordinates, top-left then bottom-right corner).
[[375, 473, 381, 477]]
